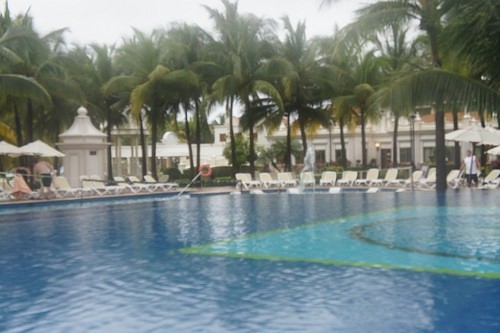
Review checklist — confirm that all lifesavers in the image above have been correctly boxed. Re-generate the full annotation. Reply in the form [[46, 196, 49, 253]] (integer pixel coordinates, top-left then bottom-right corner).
[[199, 163, 212, 177]]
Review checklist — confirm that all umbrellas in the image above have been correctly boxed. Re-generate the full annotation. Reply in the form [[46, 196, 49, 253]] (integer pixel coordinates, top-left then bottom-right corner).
[[0, 141, 28, 154], [8, 139, 66, 190], [444, 125, 500, 158]]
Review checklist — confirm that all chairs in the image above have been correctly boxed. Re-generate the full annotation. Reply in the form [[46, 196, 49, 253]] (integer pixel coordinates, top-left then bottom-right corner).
[[0, 174, 178, 201], [235, 167, 500, 191]]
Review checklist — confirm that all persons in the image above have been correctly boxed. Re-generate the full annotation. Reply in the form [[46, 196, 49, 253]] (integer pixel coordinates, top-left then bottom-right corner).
[[31, 156, 57, 199], [457, 149, 481, 187]]
